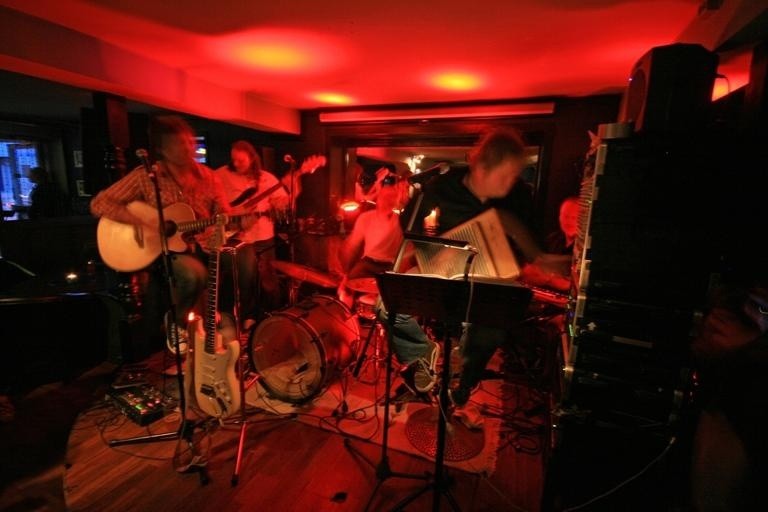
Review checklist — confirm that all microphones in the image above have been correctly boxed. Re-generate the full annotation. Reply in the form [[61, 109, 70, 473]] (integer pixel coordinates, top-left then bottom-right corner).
[[407, 162, 451, 186], [283, 154, 296, 164], [136, 148, 159, 184]]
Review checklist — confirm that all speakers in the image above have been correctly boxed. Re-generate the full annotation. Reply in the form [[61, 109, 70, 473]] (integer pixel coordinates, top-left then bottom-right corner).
[[619, 43, 720, 133]]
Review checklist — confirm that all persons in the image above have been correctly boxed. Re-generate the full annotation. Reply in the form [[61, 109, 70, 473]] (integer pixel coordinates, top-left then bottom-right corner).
[[345, 162, 422, 207], [91, 114, 229, 355], [27, 166, 73, 217], [211, 138, 299, 313], [340, 185, 405, 280], [374, 125, 553, 409]]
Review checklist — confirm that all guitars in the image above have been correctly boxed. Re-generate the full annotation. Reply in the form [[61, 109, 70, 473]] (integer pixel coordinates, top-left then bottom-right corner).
[[96, 201, 243, 272], [224, 155, 326, 242], [195, 216, 240, 420]]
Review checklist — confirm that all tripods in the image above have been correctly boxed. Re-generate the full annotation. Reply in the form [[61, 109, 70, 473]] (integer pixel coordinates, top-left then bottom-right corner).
[[215, 247, 298, 488], [343, 312, 433, 511], [108, 183, 215, 487], [389, 338, 487, 512]]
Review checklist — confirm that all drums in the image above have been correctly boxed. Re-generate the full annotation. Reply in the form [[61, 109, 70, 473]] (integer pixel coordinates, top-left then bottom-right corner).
[[246, 294, 360, 404]]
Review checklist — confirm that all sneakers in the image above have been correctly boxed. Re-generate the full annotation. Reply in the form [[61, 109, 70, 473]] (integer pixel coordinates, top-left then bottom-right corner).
[[164, 311, 188, 354], [449, 350, 469, 406], [415, 344, 440, 391]]
